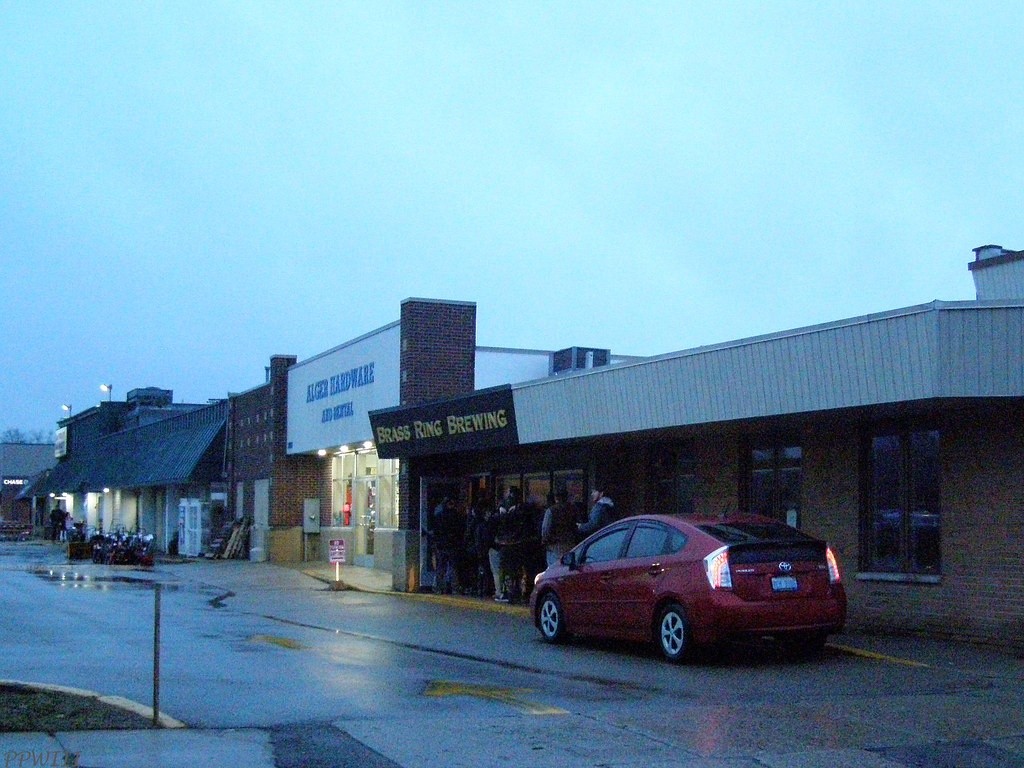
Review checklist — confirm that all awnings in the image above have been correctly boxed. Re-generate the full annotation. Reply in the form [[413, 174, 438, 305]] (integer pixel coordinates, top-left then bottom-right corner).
[[15, 420, 225, 500]]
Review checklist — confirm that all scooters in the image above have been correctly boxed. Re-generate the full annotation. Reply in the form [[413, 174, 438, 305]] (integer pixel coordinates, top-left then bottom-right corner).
[[91, 523, 156, 566], [67, 520, 87, 542]]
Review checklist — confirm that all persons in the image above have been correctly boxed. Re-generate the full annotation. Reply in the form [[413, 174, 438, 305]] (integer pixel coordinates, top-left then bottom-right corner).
[[50, 505, 70, 541], [432, 482, 615, 602]]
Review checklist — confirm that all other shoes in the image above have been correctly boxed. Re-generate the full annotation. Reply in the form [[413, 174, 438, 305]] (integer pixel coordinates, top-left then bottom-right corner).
[[495, 593, 510, 601]]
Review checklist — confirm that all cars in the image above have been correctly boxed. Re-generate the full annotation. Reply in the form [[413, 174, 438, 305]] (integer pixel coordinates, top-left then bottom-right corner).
[[531, 513, 846, 663]]
